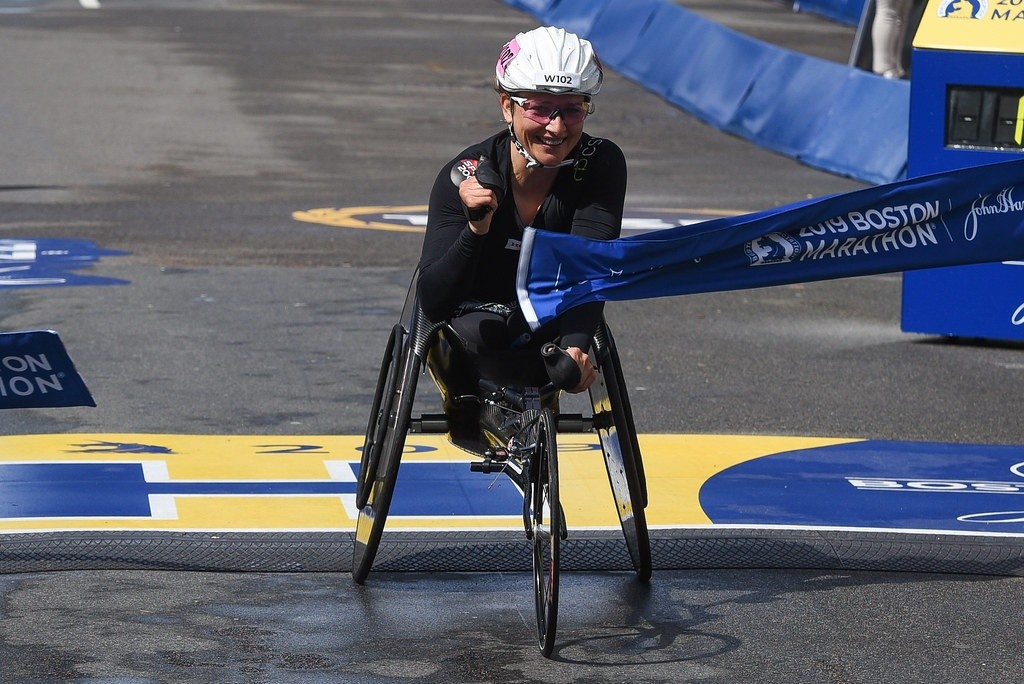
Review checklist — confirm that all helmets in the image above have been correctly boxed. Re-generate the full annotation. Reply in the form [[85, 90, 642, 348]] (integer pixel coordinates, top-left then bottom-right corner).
[[495, 25, 606, 99]]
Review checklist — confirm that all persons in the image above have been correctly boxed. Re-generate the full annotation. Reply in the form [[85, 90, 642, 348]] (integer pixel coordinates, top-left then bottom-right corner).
[[416, 27, 630, 430], [871, 0, 915, 80]]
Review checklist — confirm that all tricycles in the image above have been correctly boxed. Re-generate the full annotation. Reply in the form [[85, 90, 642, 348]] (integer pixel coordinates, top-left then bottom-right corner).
[[352, 258, 654, 658]]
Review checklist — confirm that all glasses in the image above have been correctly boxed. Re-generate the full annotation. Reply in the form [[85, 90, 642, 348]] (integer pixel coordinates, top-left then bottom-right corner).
[[505, 89, 595, 126]]
